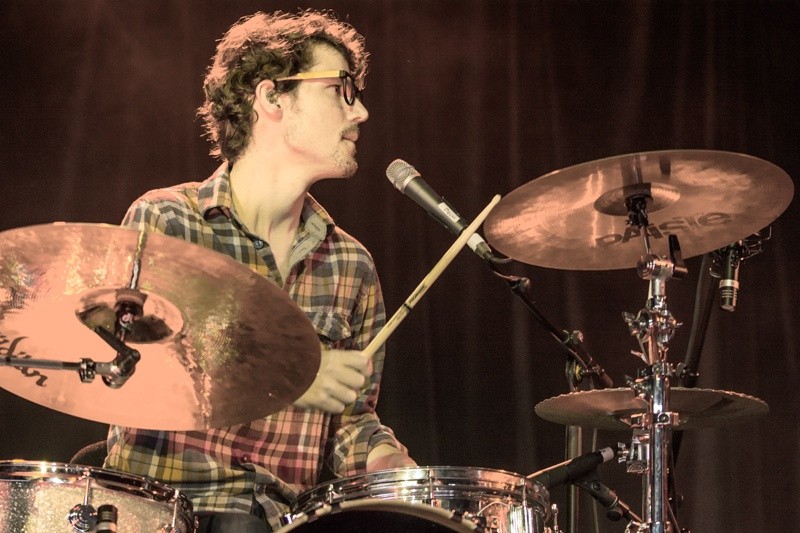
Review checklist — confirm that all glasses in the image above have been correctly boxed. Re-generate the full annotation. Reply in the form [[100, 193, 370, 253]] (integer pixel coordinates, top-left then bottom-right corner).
[[275, 69, 364, 106]]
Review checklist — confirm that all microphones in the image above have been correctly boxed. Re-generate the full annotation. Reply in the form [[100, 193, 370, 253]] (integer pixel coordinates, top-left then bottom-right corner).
[[529, 441, 629, 499], [385, 158, 494, 264]]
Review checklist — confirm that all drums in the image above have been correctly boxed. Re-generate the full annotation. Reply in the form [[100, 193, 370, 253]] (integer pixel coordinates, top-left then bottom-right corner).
[[288, 464, 559, 533], [0, 458, 200, 533], [277, 497, 487, 533]]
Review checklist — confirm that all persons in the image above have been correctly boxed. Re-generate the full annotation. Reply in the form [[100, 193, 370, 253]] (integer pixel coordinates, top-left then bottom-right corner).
[[101, 9, 420, 533]]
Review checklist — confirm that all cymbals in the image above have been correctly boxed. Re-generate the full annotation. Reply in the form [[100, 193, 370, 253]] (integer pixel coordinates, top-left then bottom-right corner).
[[0, 220, 322, 432], [483, 150, 794, 271], [533, 388, 770, 430]]
